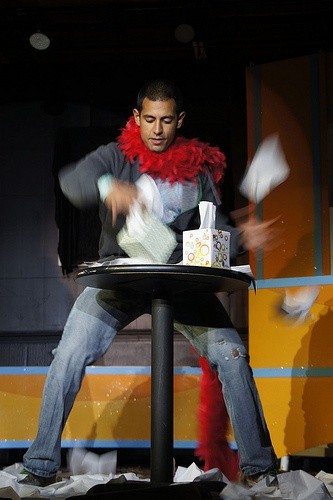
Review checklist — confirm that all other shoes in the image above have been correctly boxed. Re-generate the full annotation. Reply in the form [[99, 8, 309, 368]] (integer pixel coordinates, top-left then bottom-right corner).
[[246, 468, 285, 490], [16, 469, 62, 488]]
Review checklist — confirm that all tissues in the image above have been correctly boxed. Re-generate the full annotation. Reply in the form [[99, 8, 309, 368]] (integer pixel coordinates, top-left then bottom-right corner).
[[182, 200, 230, 270]]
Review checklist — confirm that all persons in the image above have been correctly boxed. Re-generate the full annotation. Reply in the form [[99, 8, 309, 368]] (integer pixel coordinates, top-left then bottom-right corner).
[[15, 79, 297, 497]]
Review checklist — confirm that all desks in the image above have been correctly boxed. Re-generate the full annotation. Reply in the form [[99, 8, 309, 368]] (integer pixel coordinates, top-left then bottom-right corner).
[[58, 263, 253, 500]]
